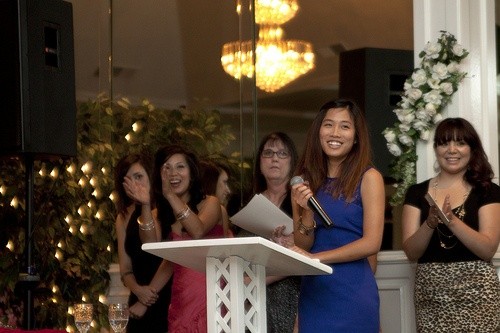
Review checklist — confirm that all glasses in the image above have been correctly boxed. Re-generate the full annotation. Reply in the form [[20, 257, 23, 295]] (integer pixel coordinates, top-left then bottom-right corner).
[[260, 149, 292, 159]]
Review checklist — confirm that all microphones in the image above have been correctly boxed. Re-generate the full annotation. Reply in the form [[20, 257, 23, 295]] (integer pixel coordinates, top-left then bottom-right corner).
[[291, 176, 334, 229]]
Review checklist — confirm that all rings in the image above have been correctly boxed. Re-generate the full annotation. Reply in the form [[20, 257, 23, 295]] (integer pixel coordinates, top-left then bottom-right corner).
[[151, 297, 156, 301]]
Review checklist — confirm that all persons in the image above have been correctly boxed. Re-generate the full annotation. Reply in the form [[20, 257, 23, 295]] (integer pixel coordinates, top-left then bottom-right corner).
[[123, 144, 224, 333], [198, 159, 233, 238], [403, 117, 500, 333], [291, 98, 385, 333], [236, 131, 300, 333], [114, 153, 174, 333]]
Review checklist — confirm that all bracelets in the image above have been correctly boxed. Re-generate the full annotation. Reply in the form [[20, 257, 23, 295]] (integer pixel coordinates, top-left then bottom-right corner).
[[426, 220, 436, 231], [121, 272, 135, 287], [137, 218, 155, 230], [296, 216, 316, 235], [176, 204, 190, 221]]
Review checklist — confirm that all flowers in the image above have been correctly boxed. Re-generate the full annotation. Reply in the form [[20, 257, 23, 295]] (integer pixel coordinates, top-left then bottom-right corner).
[[386, 31, 474, 207]]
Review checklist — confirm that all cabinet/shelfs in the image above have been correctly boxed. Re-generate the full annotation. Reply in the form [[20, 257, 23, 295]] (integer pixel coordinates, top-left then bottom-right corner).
[[374, 249, 500, 333]]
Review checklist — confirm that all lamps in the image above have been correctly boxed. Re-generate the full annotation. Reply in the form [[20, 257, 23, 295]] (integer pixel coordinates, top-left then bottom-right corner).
[[221, 0, 314, 94]]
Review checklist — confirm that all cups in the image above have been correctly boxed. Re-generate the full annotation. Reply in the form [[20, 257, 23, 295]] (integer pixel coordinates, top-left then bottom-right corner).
[[108, 303, 130, 333], [73, 303, 93, 333]]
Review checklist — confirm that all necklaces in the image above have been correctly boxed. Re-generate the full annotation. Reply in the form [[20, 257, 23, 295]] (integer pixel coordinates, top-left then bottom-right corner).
[[433, 175, 467, 248]]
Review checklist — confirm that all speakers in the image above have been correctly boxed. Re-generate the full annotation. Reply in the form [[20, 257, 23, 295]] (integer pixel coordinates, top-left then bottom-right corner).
[[339, 47, 413, 178], [0, 0, 78, 158]]
